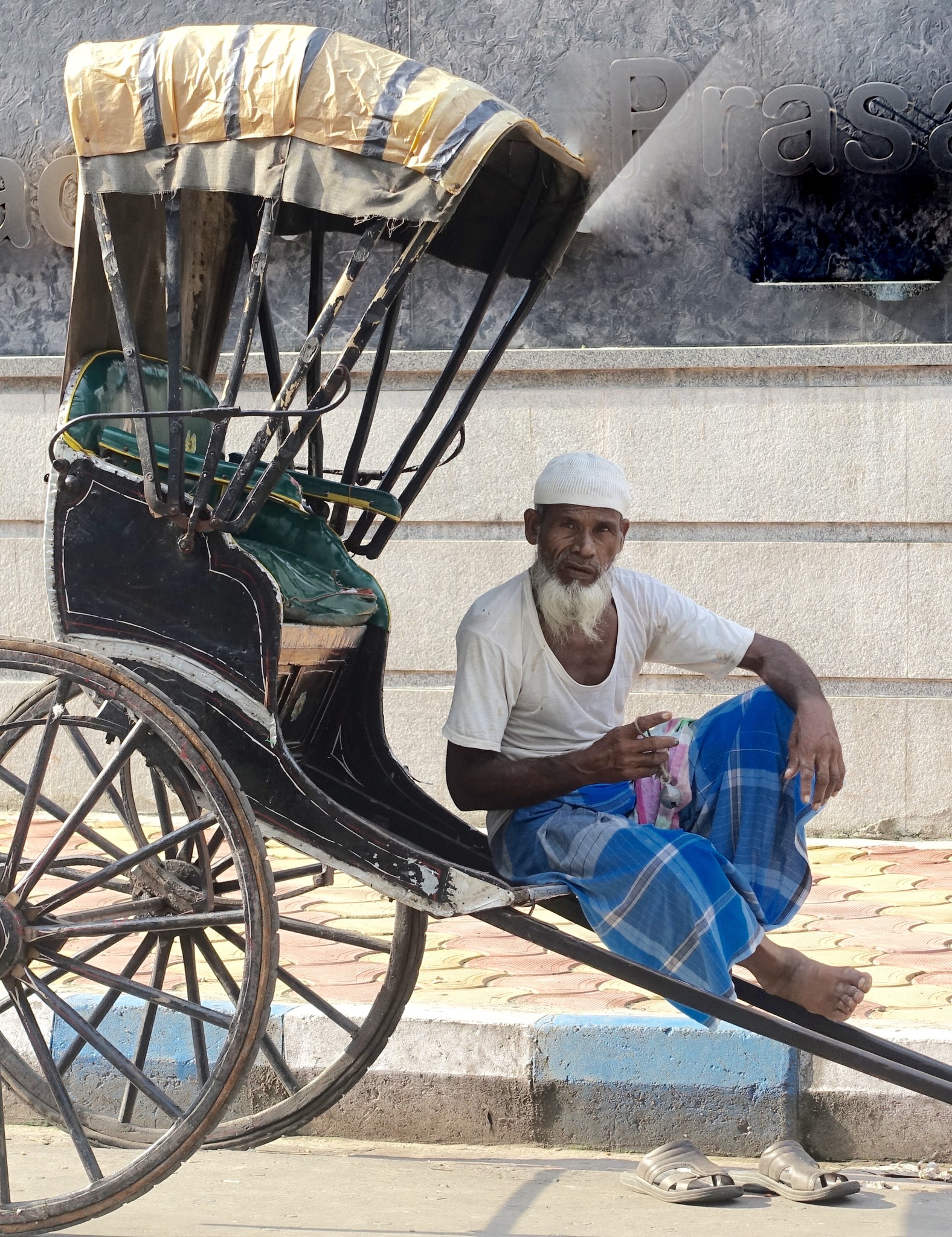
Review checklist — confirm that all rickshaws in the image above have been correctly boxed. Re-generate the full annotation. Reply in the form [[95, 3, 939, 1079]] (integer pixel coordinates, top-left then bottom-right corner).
[[1, 23, 950, 1236]]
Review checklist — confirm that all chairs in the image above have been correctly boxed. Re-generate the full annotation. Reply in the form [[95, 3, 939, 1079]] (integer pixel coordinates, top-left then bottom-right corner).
[[51, 347, 406, 672]]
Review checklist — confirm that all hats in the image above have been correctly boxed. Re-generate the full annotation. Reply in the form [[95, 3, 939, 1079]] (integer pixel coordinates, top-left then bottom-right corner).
[[534, 451, 626, 516]]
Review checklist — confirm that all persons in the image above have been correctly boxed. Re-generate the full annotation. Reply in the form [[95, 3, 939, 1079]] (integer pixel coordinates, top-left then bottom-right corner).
[[443, 452, 873, 1029]]
